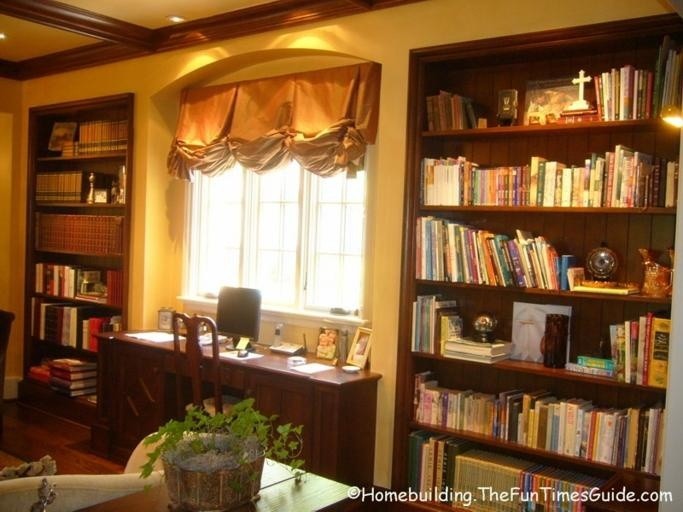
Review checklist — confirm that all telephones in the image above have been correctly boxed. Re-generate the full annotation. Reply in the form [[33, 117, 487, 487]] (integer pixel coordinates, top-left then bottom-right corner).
[[268, 324, 304, 356]]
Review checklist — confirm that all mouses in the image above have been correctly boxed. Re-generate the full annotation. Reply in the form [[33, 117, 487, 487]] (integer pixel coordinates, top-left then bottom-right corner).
[[238, 351, 248, 357]]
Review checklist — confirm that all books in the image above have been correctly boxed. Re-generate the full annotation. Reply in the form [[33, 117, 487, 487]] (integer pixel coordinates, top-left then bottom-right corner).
[[25, 115, 127, 404], [407, 38, 681, 510]]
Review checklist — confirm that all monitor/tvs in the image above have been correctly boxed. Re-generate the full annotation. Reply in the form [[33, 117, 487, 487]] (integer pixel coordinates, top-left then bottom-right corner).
[[216, 287, 262, 350]]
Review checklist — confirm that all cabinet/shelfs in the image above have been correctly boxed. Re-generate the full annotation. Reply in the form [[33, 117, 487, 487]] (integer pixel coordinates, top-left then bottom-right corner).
[[18, 94, 133, 428], [389, 9, 683, 512]]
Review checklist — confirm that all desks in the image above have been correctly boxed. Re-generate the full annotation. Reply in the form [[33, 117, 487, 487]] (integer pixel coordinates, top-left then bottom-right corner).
[[75, 459, 363, 512], [90, 329, 383, 493]]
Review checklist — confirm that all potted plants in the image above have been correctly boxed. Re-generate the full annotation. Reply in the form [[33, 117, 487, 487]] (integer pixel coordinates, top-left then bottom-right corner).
[[137, 397, 308, 509]]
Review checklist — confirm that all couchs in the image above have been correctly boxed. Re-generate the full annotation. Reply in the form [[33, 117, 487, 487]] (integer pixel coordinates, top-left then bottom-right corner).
[[0, 430, 228, 512]]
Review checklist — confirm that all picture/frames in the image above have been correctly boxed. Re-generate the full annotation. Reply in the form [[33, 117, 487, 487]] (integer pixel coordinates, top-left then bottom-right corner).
[[313, 328, 339, 365], [524, 78, 578, 126], [158, 309, 177, 332], [346, 327, 373, 370]]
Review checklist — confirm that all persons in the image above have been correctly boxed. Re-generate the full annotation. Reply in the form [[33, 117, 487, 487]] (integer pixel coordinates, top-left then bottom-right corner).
[[324, 329, 337, 359], [316, 334, 328, 359]]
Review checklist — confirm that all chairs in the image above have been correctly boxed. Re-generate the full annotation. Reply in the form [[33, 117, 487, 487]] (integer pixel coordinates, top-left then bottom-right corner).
[[171, 314, 243, 420]]
